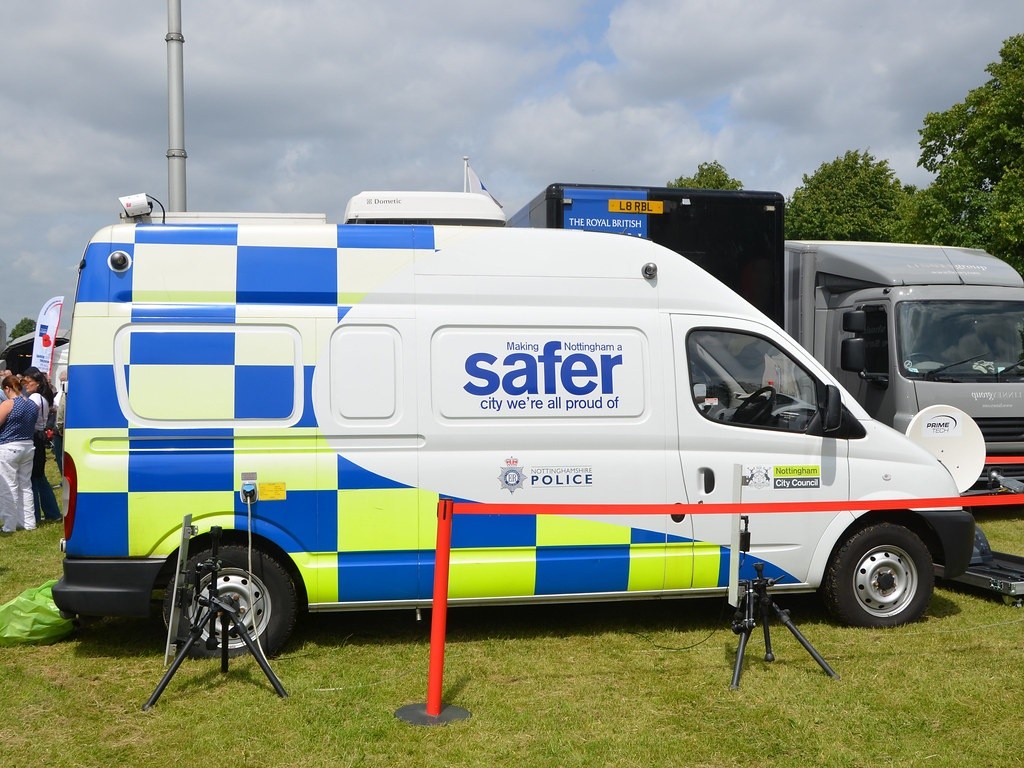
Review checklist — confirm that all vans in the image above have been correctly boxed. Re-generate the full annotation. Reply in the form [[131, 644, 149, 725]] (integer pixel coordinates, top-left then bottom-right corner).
[[46, 186, 977, 662]]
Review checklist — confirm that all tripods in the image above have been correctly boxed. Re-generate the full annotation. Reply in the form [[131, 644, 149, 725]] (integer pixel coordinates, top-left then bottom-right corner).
[[726, 562, 841, 692], [138, 526, 288, 712]]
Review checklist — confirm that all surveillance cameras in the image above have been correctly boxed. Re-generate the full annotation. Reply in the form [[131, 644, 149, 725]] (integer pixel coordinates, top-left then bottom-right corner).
[[117, 193, 153, 220]]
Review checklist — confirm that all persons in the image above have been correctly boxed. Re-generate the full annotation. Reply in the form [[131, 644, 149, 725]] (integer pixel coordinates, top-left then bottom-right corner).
[[0, 369, 29, 404], [24, 372, 65, 527], [56, 392, 66, 438], [44, 371, 68, 490], [0, 375, 42, 534]]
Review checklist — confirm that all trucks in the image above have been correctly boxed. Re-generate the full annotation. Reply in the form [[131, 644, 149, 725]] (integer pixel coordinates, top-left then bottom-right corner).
[[501, 184, 787, 330], [783, 238, 1024, 496]]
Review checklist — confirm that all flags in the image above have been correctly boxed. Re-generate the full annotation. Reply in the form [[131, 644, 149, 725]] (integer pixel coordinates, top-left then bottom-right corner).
[[30, 295, 66, 378]]
